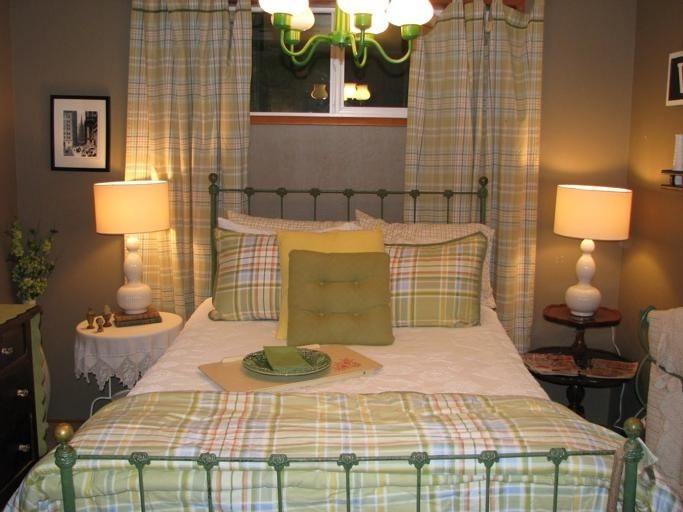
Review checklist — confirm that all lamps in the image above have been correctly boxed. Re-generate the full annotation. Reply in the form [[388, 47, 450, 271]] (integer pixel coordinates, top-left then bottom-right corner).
[[92, 181, 173, 314], [310, 74, 371, 103], [552, 185, 632, 318], [258, 0, 433, 68]]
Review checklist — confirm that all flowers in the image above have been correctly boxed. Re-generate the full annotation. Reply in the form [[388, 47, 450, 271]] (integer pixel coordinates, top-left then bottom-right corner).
[[5, 212, 60, 304]]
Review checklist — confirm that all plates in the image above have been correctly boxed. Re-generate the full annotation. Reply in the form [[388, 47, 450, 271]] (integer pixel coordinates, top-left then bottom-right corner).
[[244, 348, 332, 376]]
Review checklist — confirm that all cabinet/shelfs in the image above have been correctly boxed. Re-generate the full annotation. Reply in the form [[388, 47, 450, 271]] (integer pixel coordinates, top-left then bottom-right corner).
[[0, 303, 42, 512], [518, 304, 636, 418]]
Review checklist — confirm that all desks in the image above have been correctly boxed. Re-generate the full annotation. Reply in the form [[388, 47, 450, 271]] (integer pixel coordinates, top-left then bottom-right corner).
[[73, 313, 186, 417]]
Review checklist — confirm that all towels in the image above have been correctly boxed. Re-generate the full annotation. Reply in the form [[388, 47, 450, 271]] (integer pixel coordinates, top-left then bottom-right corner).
[[263, 345, 313, 372]]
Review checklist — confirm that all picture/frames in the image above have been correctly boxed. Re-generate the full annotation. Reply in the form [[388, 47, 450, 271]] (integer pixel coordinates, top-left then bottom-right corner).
[[663, 50, 683, 107], [49, 95, 110, 172]]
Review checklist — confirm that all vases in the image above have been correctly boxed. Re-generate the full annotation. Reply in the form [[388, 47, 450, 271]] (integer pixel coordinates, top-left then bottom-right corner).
[[21, 295, 52, 456]]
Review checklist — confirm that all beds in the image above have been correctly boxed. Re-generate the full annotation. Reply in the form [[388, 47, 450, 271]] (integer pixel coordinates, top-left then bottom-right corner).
[[4, 174, 683, 511]]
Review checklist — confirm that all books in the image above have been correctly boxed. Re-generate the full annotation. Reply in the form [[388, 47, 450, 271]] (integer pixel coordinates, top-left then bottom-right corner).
[[521, 350, 639, 380], [112, 307, 162, 328]]
